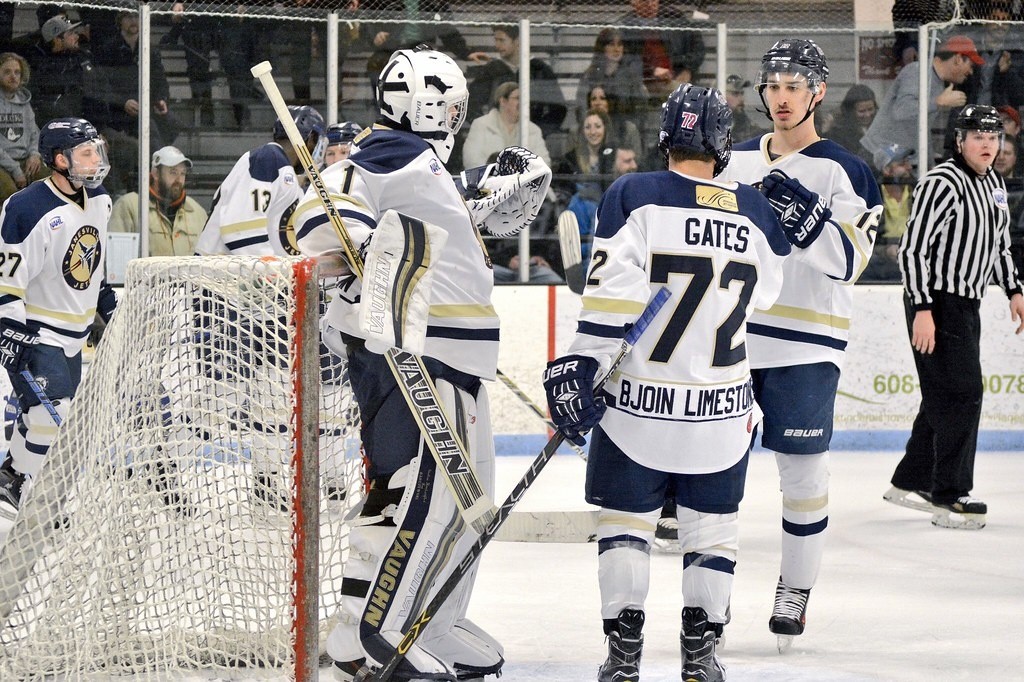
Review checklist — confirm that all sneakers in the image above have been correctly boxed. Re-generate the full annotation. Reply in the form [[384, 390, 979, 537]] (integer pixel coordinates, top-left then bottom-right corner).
[[0, 461, 70, 531], [769, 576, 811, 653], [652, 512, 683, 554], [930, 491, 989, 529], [677, 607, 728, 681], [880, 465, 934, 513], [596, 608, 646, 682]]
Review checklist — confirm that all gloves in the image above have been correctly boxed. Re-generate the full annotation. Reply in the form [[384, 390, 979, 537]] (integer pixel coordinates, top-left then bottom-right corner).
[[544, 350, 612, 448], [0, 316, 40, 374], [755, 167, 833, 252], [93, 289, 118, 334]]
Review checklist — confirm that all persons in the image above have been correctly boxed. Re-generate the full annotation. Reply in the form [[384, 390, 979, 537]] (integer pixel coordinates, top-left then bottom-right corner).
[[542, 84, 793, 682], [722, 37, 884, 656], [290, 44, 556, 682], [0, 0, 1024, 532]]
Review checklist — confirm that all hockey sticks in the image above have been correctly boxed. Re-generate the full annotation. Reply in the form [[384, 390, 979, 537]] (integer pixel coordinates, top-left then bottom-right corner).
[[248, 57, 604, 546], [23, 365, 134, 483], [370, 283, 673, 679], [494, 368, 594, 466], [180, 400, 249, 443], [556, 204, 586, 297]]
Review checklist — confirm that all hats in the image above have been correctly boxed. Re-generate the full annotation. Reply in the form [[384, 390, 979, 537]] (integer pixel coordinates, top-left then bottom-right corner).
[[872, 143, 916, 174], [152, 147, 193, 169], [996, 105, 1020, 126], [41, 15, 83, 39], [495, 82, 520, 102], [937, 35, 985, 66]]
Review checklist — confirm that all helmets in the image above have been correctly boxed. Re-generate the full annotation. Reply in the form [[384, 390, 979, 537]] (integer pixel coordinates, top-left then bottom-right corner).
[[271, 103, 329, 175], [954, 102, 1002, 131], [38, 118, 111, 190], [327, 120, 360, 146], [661, 83, 737, 177], [760, 39, 831, 82], [377, 44, 469, 165]]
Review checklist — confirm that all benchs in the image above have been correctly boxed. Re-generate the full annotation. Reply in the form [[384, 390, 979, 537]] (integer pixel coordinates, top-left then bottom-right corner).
[[12, 0, 952, 243]]
